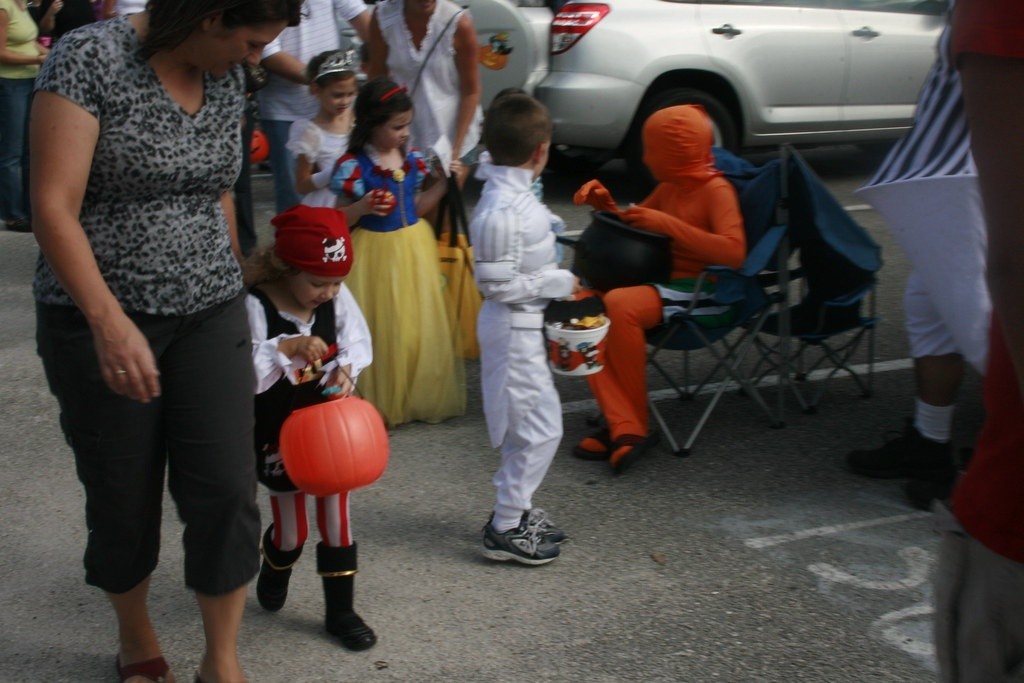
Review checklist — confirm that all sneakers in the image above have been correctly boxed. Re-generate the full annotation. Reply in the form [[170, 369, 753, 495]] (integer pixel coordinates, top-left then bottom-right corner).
[[845, 432, 949, 479], [520, 510, 562, 544], [480, 523, 557, 565]]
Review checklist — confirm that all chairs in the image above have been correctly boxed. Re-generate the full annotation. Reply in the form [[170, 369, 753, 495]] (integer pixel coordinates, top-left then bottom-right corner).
[[642, 143, 884, 459]]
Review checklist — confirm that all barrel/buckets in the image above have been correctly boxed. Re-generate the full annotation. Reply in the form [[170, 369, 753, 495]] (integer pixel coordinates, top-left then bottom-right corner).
[[545, 314, 611, 376], [572, 209, 672, 291]]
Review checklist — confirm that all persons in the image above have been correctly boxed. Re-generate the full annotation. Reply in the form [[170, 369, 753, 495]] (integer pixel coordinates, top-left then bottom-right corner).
[[850, 0, 1024, 683], [0, 0, 147, 231], [467, 88, 583, 565], [572, 104, 749, 472], [237, 0, 481, 429], [27, 0, 304, 683], [243, 204, 378, 651]]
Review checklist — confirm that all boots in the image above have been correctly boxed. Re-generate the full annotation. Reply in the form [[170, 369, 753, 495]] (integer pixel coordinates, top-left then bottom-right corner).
[[257, 522, 304, 612], [316, 541, 378, 651]]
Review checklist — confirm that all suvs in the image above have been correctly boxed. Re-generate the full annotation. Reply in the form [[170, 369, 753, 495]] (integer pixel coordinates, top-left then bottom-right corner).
[[455, 0, 948, 204]]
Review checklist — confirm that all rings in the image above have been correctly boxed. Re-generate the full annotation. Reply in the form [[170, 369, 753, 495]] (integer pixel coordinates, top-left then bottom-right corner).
[[116, 370, 126, 374]]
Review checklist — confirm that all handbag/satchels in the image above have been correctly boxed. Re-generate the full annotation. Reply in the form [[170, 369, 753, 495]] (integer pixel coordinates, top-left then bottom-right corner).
[[435, 174, 485, 360]]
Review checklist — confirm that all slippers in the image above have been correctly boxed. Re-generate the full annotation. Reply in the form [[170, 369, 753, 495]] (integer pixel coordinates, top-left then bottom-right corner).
[[574, 430, 616, 457], [608, 428, 661, 472], [116, 649, 171, 683]]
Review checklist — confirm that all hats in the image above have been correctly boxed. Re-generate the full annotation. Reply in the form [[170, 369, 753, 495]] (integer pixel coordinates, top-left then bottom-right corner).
[[271, 204, 354, 275]]
